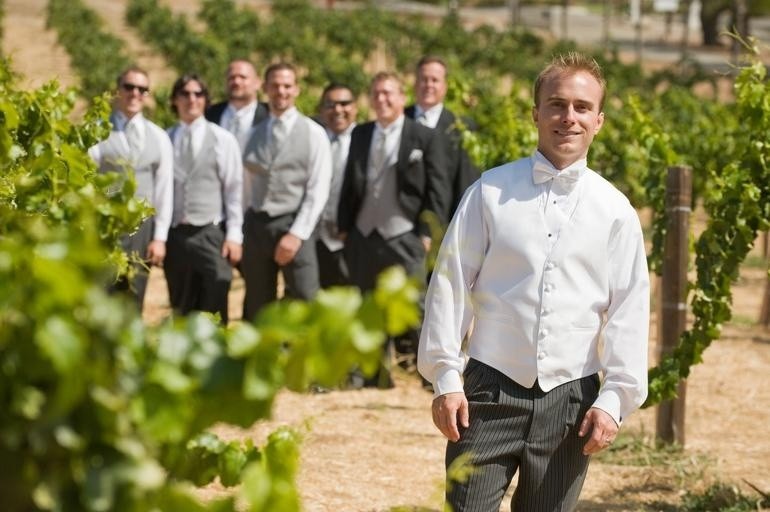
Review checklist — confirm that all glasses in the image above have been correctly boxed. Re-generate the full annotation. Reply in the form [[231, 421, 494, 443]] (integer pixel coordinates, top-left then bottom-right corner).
[[180, 91, 204, 98], [123, 85, 150, 95]]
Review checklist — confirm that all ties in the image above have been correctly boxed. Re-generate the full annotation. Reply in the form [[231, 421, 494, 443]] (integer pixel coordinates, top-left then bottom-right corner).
[[377, 131, 389, 164], [125, 122, 137, 148], [329, 134, 339, 163], [415, 115, 424, 124], [183, 131, 194, 172], [231, 117, 241, 138], [271, 119, 286, 156]]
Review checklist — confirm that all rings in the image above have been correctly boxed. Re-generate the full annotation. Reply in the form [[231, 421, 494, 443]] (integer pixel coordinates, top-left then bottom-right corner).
[[605, 439, 611, 442]]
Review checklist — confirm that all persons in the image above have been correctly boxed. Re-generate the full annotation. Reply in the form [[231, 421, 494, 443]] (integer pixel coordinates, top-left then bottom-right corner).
[[236, 62, 332, 341], [401, 55, 485, 222], [414, 52, 650, 510], [82, 67, 173, 323], [204, 57, 269, 137], [335, 71, 450, 392], [158, 73, 244, 336], [310, 82, 360, 288]]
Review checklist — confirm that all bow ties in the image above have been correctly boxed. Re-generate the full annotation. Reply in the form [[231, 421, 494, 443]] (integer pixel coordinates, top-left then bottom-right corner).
[[533, 161, 579, 196]]
